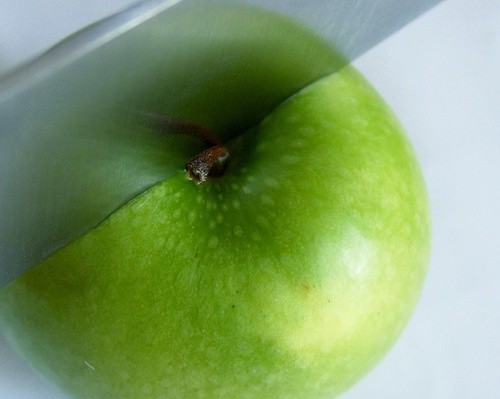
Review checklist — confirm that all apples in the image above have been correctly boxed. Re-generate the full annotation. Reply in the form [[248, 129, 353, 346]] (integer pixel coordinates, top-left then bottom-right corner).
[[0, 59, 434, 399], [2, 0, 346, 278]]
